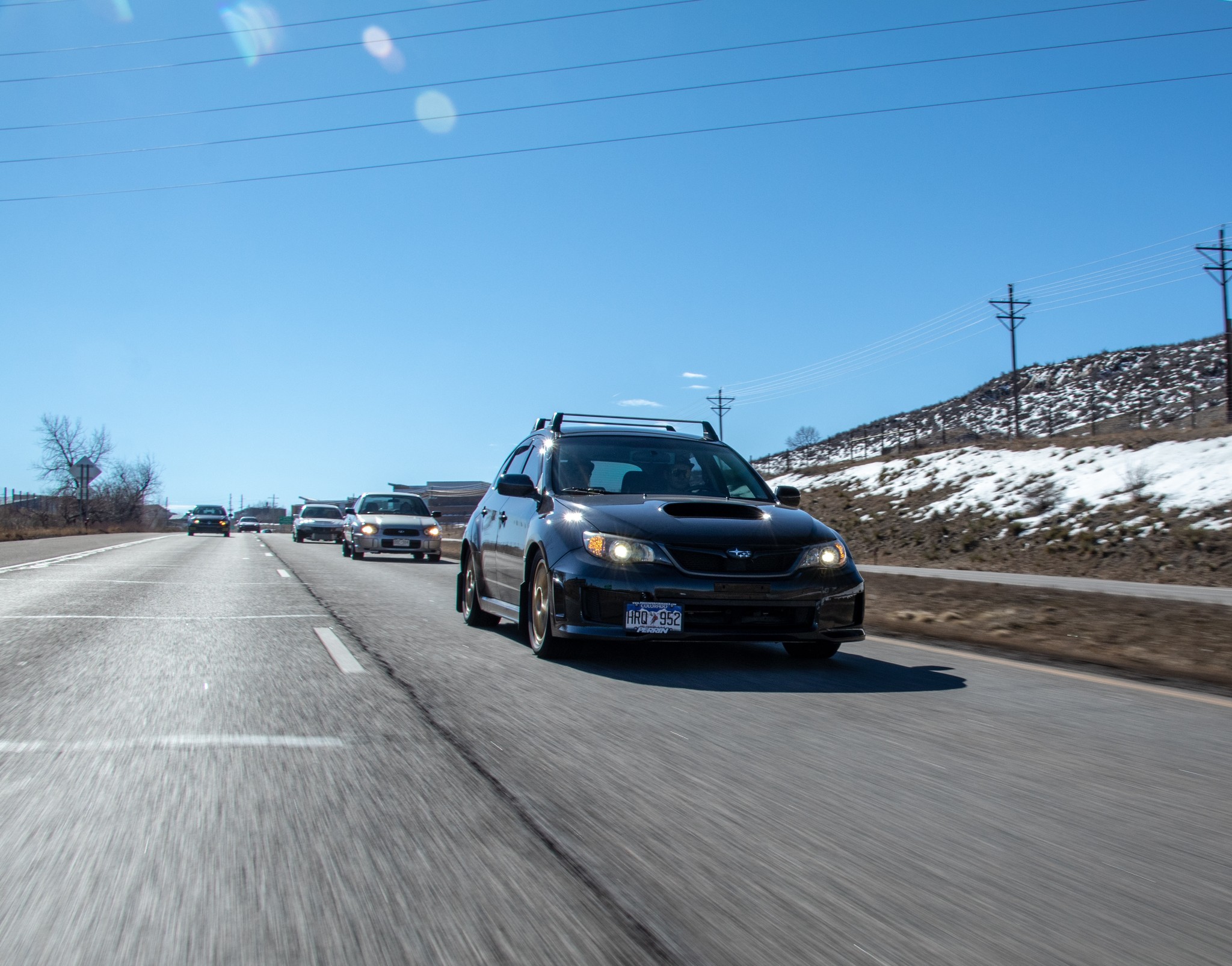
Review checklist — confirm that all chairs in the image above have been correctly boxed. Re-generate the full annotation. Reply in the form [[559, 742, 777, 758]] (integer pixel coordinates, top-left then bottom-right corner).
[[619, 471, 668, 494], [364, 502, 378, 513]]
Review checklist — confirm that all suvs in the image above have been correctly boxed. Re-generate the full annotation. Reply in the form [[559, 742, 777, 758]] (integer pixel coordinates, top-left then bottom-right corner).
[[457, 412, 868, 660]]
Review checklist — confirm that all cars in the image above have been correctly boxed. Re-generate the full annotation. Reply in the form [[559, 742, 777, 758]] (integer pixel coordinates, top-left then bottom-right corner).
[[342, 492, 443, 563], [235, 516, 262, 533], [293, 503, 346, 544], [186, 504, 233, 538]]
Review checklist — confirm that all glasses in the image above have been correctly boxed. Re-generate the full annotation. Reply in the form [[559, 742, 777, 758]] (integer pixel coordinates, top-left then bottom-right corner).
[[670, 469, 691, 478], [567, 462, 592, 477]]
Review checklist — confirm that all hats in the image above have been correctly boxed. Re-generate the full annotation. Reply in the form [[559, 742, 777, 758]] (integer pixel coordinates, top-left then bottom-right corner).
[[562, 454, 595, 475], [667, 456, 695, 470]]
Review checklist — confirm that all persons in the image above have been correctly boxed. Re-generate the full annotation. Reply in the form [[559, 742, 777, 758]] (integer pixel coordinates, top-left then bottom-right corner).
[[563, 455, 595, 490], [659, 456, 694, 496], [401, 502, 411, 513]]
